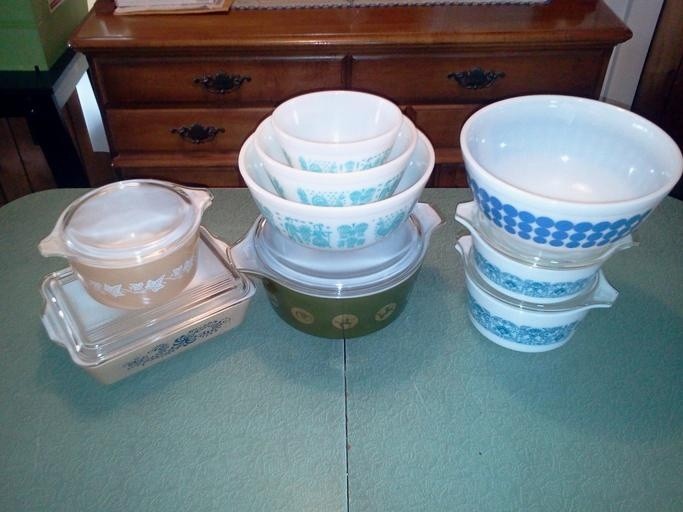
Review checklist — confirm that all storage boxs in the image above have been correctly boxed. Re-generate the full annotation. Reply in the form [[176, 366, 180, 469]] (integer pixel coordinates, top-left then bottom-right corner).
[[0, 2, 88, 71]]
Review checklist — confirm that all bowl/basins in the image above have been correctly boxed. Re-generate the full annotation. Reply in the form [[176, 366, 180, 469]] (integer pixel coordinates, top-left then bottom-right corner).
[[238, 132, 437, 250], [39, 178, 214, 312], [226, 200, 447, 339], [459, 94, 681, 253], [39, 225, 256, 383], [257, 118, 417, 206], [454, 201, 642, 306], [271, 90, 400, 172], [454, 233, 621, 353]]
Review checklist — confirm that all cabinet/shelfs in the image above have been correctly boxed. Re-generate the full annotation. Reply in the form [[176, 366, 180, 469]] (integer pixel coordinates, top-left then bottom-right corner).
[[67, 0, 635, 188], [0, 50, 109, 208]]
